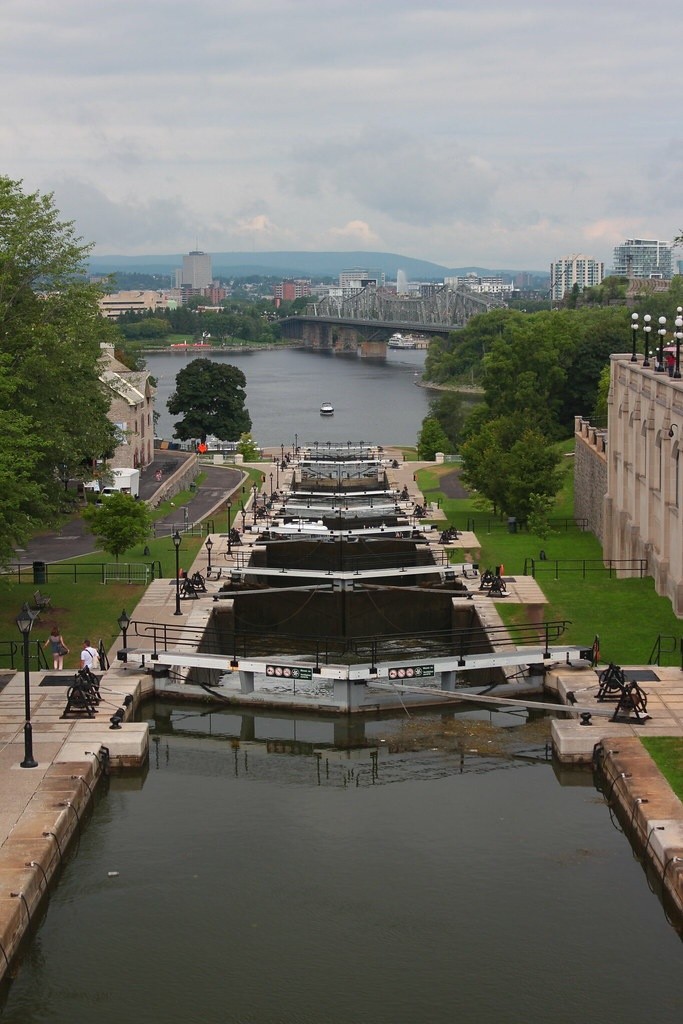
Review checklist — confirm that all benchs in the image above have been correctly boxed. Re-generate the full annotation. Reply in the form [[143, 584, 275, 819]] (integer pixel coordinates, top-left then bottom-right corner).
[[21, 602, 41, 625], [33, 590, 51, 611]]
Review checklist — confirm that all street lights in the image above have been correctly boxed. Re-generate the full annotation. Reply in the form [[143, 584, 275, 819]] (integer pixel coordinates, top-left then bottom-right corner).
[[276, 458, 280, 489], [643, 314, 651, 366], [116, 609, 132, 663], [269, 472, 273, 503], [657, 316, 666, 371], [251, 482, 258, 525], [13, 602, 40, 768], [292, 443, 295, 463], [205, 536, 214, 577], [226, 497, 232, 554], [631, 312, 638, 361], [295, 433, 299, 455], [241, 507, 247, 534], [172, 531, 182, 615], [674, 307, 683, 378], [281, 444, 284, 472]]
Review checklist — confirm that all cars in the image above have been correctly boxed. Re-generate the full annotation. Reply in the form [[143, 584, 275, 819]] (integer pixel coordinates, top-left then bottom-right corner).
[[96, 487, 123, 506]]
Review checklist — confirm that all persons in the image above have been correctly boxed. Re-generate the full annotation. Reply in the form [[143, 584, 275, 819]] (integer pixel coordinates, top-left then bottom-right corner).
[[287, 452, 291, 463], [401, 484, 429, 516], [80, 640, 102, 672], [43, 625, 69, 671], [251, 501, 259, 510]]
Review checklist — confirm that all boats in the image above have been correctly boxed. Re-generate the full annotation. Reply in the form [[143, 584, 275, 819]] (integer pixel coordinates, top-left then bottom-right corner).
[[320, 401, 334, 416], [388, 333, 415, 349]]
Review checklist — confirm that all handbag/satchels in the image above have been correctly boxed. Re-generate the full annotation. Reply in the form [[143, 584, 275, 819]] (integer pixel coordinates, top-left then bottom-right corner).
[[93, 656, 100, 669], [58, 646, 68, 656]]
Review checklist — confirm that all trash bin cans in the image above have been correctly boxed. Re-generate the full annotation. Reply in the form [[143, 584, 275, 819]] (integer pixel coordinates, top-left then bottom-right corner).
[[32, 561, 46, 584], [507, 518, 517, 533], [190, 482, 196, 492]]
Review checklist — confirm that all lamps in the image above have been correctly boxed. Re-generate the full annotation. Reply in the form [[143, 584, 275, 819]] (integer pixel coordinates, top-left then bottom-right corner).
[[669, 424, 678, 437]]
